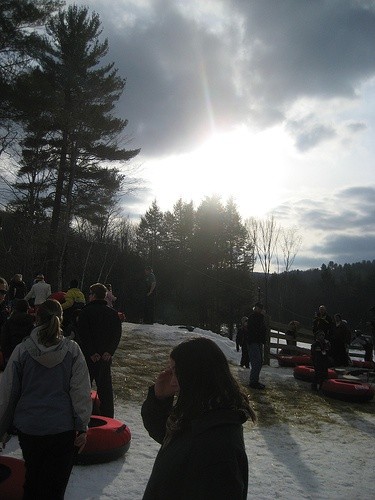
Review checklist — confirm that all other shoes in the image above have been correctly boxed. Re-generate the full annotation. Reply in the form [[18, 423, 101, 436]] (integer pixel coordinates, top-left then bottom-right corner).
[[250, 382, 265, 390]]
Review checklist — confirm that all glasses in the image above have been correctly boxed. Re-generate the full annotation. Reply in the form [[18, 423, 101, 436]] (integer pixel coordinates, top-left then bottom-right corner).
[[88, 292, 92, 296], [0, 290, 8, 295]]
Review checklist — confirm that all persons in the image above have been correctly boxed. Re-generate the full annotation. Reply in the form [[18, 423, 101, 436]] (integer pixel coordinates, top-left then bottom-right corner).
[[0, 272, 118, 368], [140, 337, 255, 500], [142, 266, 157, 324], [236, 301, 372, 395], [73, 283, 122, 418], [0, 298, 92, 500]]
